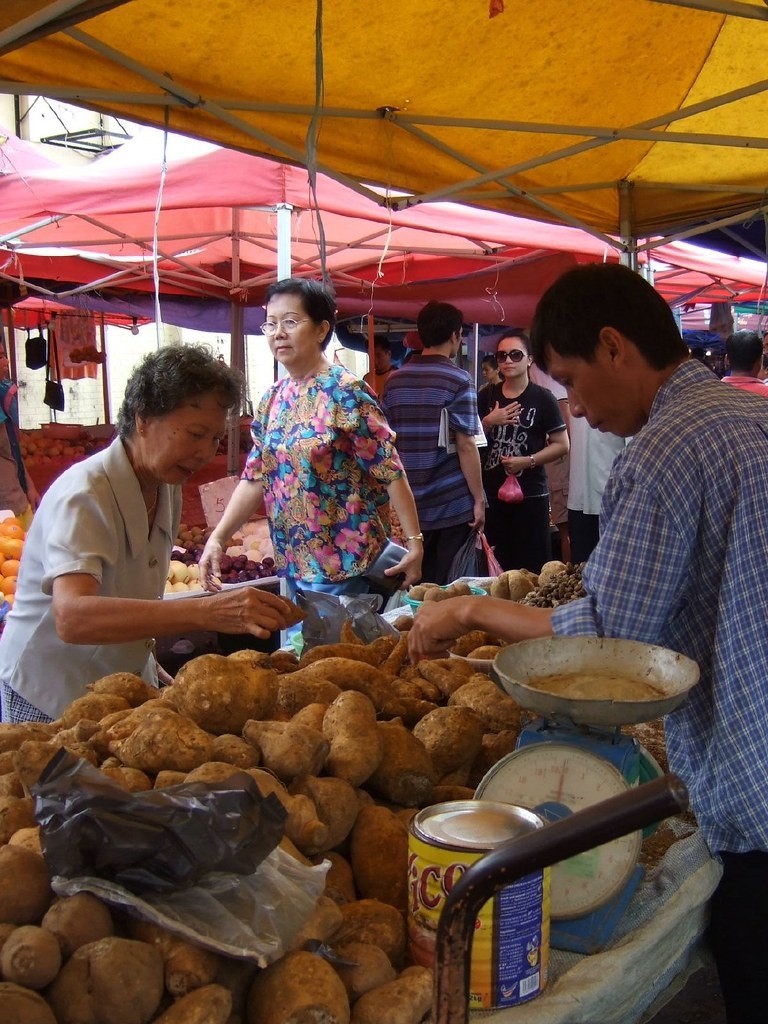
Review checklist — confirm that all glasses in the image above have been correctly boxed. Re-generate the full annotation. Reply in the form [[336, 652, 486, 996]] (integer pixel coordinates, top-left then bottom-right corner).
[[494, 350, 529, 363], [260, 319, 314, 336]]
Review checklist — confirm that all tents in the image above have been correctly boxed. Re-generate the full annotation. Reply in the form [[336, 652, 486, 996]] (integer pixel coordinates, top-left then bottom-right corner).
[[0, 0, 768, 273]]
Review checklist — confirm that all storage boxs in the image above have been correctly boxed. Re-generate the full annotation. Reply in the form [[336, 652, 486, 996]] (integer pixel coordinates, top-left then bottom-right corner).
[[162, 575, 296, 650]]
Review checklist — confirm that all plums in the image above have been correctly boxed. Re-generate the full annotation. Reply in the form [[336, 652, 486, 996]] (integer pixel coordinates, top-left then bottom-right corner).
[[172, 548, 280, 584]]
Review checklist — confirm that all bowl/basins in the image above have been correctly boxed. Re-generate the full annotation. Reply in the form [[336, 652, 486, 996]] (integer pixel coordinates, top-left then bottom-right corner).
[[403, 584, 488, 615]]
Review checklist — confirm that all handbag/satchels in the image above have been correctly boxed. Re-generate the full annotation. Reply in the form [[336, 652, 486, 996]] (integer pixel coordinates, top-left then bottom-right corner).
[[384, 584, 415, 613], [44, 380, 65, 411], [26, 324, 47, 370], [498, 455, 524, 503], [448, 529, 505, 584]]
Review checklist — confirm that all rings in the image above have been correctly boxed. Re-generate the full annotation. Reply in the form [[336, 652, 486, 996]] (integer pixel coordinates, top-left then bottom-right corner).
[[508, 409, 511, 413]]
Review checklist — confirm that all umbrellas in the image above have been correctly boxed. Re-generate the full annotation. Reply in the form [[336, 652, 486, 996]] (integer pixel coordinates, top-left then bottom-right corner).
[[0, 122, 768, 479]]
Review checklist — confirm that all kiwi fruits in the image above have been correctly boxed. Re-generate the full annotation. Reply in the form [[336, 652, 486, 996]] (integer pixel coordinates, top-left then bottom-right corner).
[[174, 522, 245, 552]]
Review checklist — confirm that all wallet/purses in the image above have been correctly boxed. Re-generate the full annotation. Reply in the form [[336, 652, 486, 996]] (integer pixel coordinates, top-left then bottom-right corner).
[[360, 541, 411, 595]]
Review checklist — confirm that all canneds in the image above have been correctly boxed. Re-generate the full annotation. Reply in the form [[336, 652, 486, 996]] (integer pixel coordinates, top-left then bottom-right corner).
[[407, 798, 551, 1010]]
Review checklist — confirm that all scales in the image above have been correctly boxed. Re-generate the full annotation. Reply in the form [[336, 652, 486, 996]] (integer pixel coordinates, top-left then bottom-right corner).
[[475, 635, 701, 954]]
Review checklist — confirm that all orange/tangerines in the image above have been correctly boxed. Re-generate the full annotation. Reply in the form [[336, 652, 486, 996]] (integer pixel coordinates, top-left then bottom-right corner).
[[0, 517, 27, 609]]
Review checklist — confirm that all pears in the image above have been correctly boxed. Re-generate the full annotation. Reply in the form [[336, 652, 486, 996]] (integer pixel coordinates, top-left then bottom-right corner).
[[163, 559, 223, 595], [227, 517, 272, 563]]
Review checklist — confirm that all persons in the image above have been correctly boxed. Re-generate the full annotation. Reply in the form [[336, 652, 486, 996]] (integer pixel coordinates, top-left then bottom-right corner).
[[527, 363, 570, 563], [198, 279, 424, 640], [406, 261, 768, 1024], [478, 354, 502, 392], [380, 299, 490, 588], [362, 335, 400, 398], [0, 343, 49, 513], [566, 416, 625, 566], [758, 354, 768, 384], [720, 328, 768, 398], [763, 331, 768, 355], [477, 328, 570, 577], [0, 346, 291, 725]]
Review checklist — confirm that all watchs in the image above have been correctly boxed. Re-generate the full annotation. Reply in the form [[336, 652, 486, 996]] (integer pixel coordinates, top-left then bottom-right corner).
[[407, 532, 424, 542], [530, 455, 535, 468]]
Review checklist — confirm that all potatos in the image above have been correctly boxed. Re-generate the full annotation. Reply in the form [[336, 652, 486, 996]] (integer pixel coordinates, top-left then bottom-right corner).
[[0, 612, 540, 1024], [406, 559, 568, 602]]
